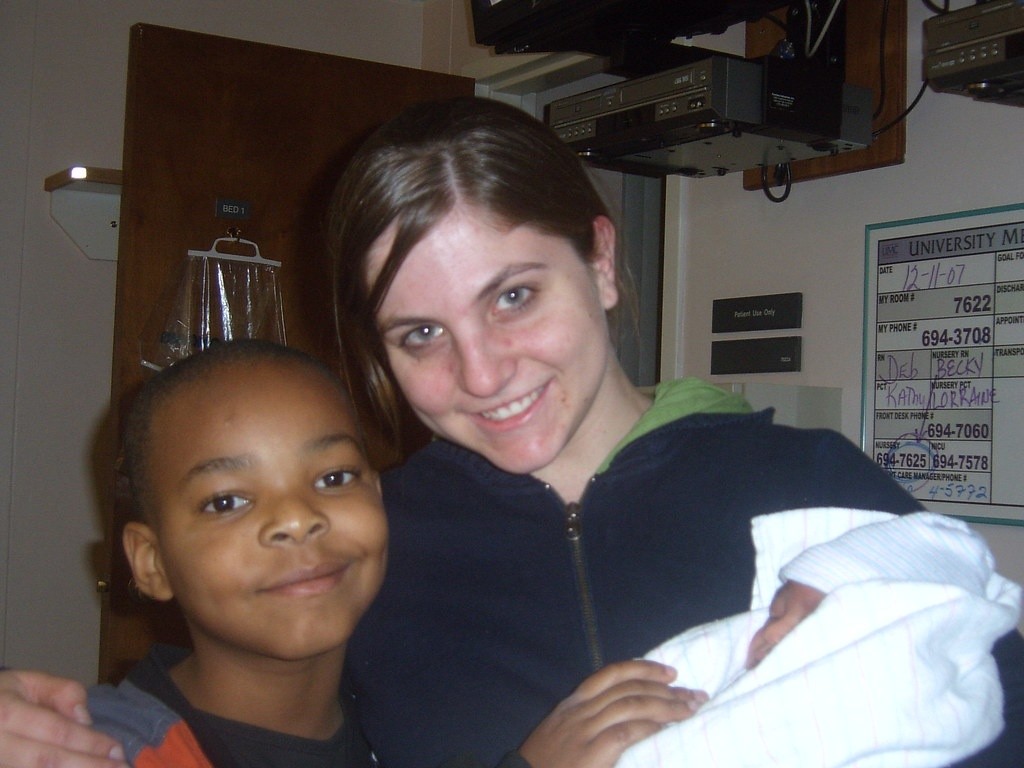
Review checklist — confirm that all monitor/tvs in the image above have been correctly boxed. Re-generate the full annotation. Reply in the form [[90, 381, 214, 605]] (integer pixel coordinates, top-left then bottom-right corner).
[[471, 0, 792, 57]]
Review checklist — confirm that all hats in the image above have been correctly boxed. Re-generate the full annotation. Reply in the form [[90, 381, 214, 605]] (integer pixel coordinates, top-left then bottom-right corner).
[[777, 509, 995, 597]]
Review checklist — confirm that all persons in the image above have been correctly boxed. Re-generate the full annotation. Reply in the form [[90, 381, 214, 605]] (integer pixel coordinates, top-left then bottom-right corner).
[[0, 94, 1024, 768], [79, 338, 710, 768], [611, 507, 1022, 768]]
